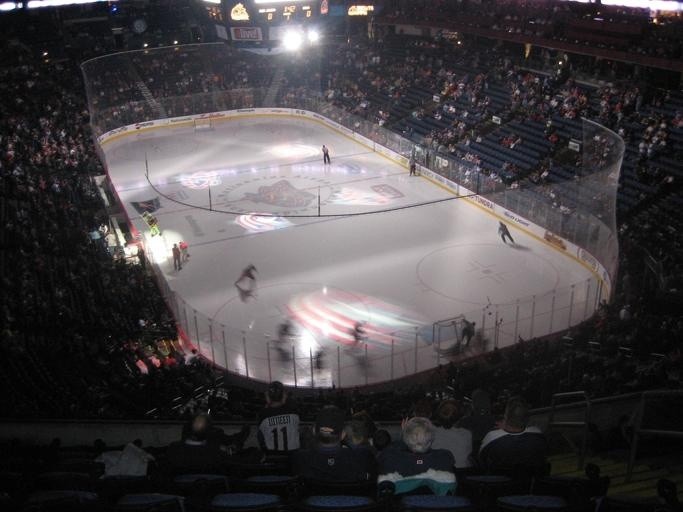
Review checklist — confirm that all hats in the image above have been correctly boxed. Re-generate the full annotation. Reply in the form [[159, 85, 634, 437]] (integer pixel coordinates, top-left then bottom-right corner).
[[314, 406, 346, 434]]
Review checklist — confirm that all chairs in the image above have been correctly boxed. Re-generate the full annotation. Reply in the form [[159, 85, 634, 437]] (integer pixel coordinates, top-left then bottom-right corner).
[[342, 48, 683, 253], [0, 445, 594, 511]]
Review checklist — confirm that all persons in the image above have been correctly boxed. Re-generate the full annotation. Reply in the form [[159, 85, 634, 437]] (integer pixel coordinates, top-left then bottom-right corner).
[[347, 321, 367, 350], [234, 263, 256, 287], [0, 0, 682, 180], [183, 350, 682, 511], [0, 182, 184, 511], [179, 242, 191, 263], [495, 179, 683, 350], [236, 285, 257, 304], [459, 317, 476, 347]]
